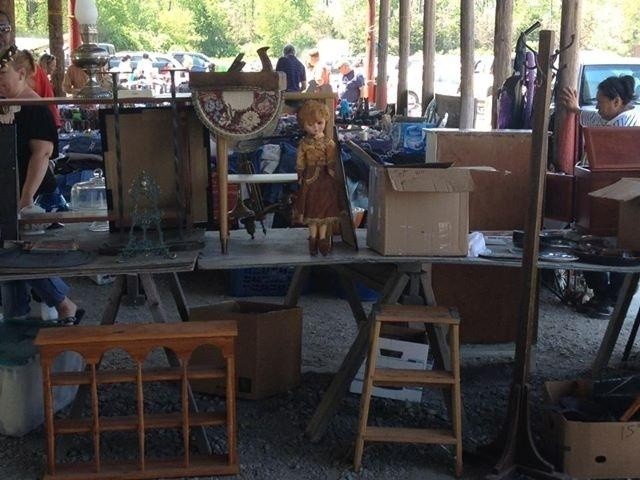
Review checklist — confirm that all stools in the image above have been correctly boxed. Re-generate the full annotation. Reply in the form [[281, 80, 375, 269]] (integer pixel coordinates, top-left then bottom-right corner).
[[353, 303, 463, 478]]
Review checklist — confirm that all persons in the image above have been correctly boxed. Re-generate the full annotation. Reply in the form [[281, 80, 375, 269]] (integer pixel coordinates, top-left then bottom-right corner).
[[177, 65, 206, 93], [38, 54, 57, 75], [120, 54, 131, 72], [139, 71, 146, 80], [129, 68, 137, 81], [1, 44, 86, 327], [136, 53, 153, 79], [160, 63, 171, 74], [306, 51, 329, 87], [294, 100, 344, 258], [560, 75, 640, 318], [0, 9, 62, 323], [305, 64, 333, 94], [276, 44, 306, 107], [339, 60, 365, 102], [63, 64, 112, 108]]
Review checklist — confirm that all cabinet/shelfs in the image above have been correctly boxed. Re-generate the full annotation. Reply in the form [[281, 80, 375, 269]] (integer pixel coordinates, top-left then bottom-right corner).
[[422, 128, 553, 380], [33, 318, 241, 476]]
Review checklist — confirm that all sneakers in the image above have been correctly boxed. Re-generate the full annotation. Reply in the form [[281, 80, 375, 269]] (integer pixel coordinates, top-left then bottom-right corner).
[[309, 236, 318, 255], [318, 238, 328, 256], [576, 296, 611, 320]]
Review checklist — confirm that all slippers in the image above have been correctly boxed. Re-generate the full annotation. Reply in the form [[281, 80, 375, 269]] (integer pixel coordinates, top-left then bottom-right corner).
[[55, 308, 86, 328]]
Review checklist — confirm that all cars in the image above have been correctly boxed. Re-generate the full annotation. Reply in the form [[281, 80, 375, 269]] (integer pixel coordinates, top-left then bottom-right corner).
[[106, 51, 183, 76], [167, 51, 215, 73], [386, 58, 422, 111], [440, 53, 518, 113], [575, 56, 640, 111]]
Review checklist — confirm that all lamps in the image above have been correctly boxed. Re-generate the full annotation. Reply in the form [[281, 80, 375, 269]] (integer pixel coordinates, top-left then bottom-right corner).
[[71, 1, 112, 98]]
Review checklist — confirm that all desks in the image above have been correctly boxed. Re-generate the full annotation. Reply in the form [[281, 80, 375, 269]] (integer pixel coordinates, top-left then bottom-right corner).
[[0, 232, 215, 462], [199, 226, 640, 443]]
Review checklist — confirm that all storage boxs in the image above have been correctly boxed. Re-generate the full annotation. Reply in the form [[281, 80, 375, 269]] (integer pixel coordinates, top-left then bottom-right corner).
[[573, 126, 640, 240], [343, 140, 511, 256], [587, 178, 640, 261], [188, 300, 303, 402], [545, 379, 640, 480]]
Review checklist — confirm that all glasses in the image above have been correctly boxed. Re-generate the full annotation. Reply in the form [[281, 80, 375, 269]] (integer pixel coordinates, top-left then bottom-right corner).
[[0, 25, 12, 33]]
